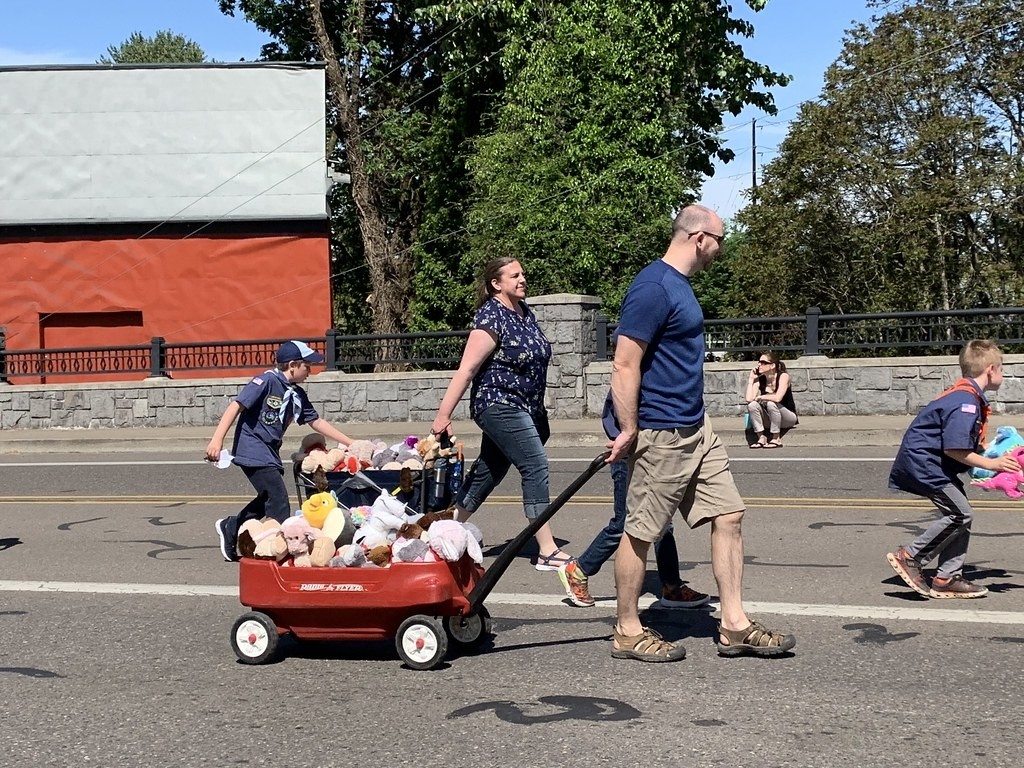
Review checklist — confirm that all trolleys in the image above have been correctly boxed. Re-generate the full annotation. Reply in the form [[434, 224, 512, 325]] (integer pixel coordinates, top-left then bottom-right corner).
[[288, 428, 490, 521], [230, 449, 618, 669]]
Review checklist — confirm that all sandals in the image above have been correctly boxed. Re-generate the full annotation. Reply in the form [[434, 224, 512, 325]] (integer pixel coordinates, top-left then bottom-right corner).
[[718, 620, 796, 656], [535, 549, 578, 571], [610, 625, 687, 663]]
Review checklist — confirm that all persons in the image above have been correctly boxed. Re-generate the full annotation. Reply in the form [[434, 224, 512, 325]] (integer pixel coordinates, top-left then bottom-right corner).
[[606, 205, 798, 663], [559, 325, 712, 609], [205, 340, 356, 561], [745, 351, 799, 449], [428, 259, 577, 572], [883, 341, 1021, 600]]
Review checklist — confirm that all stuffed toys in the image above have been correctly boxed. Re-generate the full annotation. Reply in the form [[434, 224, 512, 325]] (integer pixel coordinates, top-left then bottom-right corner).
[[970, 445, 1024, 501], [292, 429, 456, 469], [234, 489, 486, 568], [968, 426, 1023, 492]]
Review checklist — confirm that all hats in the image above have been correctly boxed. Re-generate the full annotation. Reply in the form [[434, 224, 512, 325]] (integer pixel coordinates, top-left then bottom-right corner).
[[276, 341, 326, 362]]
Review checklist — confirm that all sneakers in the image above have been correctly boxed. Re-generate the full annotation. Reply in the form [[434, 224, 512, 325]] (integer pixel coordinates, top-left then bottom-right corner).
[[559, 561, 596, 606], [886, 548, 930, 595], [215, 515, 237, 562], [661, 584, 710, 607], [929, 575, 989, 599]]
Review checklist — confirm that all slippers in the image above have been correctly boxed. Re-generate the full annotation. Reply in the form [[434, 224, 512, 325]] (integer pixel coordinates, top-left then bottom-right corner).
[[763, 442, 783, 448], [750, 442, 765, 448]]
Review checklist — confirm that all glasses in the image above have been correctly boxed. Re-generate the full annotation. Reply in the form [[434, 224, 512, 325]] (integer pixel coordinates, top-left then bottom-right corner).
[[688, 230, 724, 244], [759, 360, 773, 365]]
